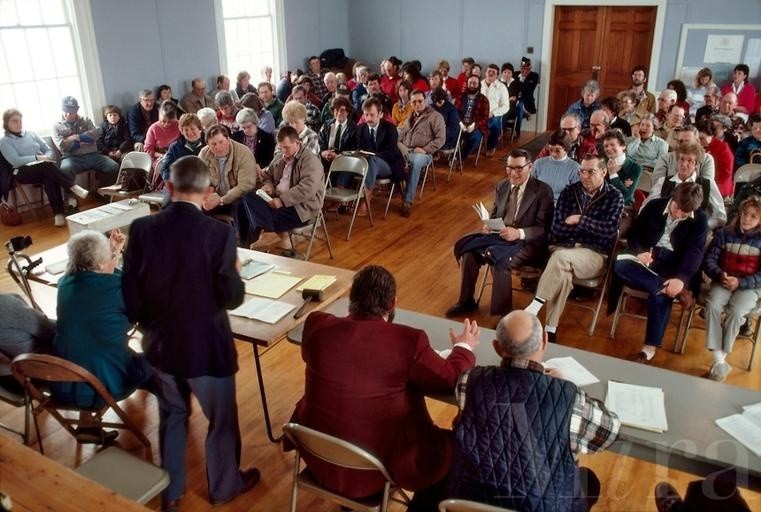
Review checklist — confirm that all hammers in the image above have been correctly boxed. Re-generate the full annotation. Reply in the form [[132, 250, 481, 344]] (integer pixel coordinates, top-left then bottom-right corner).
[[293, 288, 323, 319]]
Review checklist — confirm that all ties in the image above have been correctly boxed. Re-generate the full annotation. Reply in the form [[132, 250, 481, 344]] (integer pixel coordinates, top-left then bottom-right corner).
[[504, 187, 519, 226], [333, 125, 343, 154], [369, 128, 376, 152]]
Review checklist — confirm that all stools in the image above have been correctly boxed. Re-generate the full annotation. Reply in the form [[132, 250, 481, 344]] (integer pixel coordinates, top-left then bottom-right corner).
[[73, 445, 172, 512]]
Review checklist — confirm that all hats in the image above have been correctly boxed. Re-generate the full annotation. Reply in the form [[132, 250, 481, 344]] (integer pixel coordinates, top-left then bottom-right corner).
[[520, 57, 534, 70], [61, 96, 81, 115]]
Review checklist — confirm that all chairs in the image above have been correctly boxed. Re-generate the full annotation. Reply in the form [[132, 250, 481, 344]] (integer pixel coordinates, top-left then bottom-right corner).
[[10, 354, 156, 462], [0, 353, 45, 446], [437, 498, 513, 512], [281, 422, 412, 512], [1, 78, 528, 306], [566, 163, 761, 371]]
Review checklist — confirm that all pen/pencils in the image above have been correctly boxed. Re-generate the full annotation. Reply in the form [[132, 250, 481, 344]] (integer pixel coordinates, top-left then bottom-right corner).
[[271, 269, 291, 275], [646, 246, 653, 268]]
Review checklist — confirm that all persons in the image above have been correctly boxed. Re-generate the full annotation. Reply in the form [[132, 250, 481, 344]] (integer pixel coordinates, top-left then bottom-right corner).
[[52, 227, 159, 445], [654, 478, 749, 511], [282, 265, 480, 511], [445, 61, 759, 383], [121, 156, 261, 511], [1, 57, 541, 261], [1, 292, 57, 398], [454, 310, 622, 511]]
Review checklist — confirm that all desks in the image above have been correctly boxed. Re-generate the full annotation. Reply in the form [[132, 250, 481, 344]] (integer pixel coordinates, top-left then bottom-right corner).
[[8, 222, 358, 443], [285, 300, 761, 511], [0, 433, 158, 511]]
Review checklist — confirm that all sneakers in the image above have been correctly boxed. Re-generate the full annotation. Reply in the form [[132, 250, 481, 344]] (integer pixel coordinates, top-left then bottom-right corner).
[[709, 358, 732, 383], [328, 190, 414, 218], [53, 188, 88, 228], [445, 297, 476, 319], [250, 231, 294, 252], [655, 480, 684, 512]]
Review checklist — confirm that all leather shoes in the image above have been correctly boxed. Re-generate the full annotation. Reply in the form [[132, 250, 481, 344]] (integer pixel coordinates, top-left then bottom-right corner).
[[210, 465, 260, 506], [161, 481, 188, 510]]
